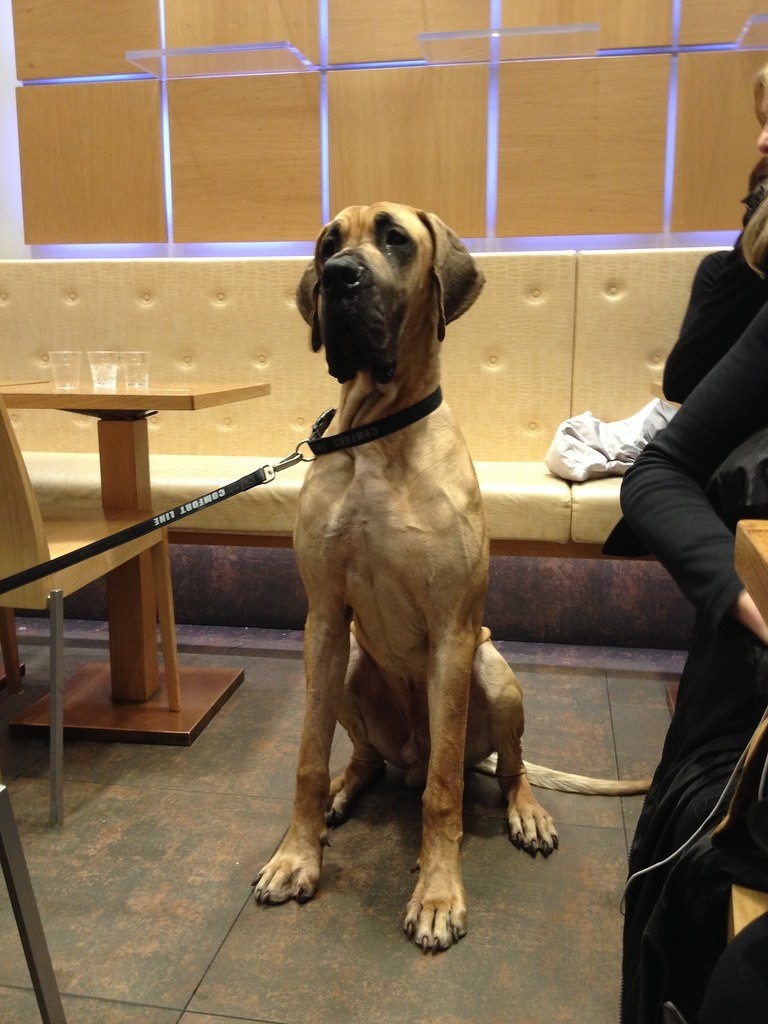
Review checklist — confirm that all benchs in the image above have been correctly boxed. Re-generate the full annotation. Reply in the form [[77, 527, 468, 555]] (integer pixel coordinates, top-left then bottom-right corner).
[[0, 247, 736, 650]]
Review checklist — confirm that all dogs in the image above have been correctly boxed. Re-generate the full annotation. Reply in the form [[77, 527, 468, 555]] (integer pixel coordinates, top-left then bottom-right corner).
[[250, 201, 656, 955]]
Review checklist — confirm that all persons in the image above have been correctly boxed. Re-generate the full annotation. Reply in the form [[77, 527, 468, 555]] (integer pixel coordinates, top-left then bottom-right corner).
[[618, 64, 768, 1024]]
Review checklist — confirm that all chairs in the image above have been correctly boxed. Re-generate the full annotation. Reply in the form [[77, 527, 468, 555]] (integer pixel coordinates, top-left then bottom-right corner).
[[1, 396, 182, 829]]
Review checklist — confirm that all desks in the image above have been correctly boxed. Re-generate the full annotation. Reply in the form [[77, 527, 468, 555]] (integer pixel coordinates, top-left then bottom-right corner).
[[1, 379, 273, 750]]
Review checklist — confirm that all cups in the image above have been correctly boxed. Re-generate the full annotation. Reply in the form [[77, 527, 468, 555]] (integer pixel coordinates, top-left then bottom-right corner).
[[88, 351, 119, 391], [120, 352, 149, 391], [49, 351, 82, 389]]
